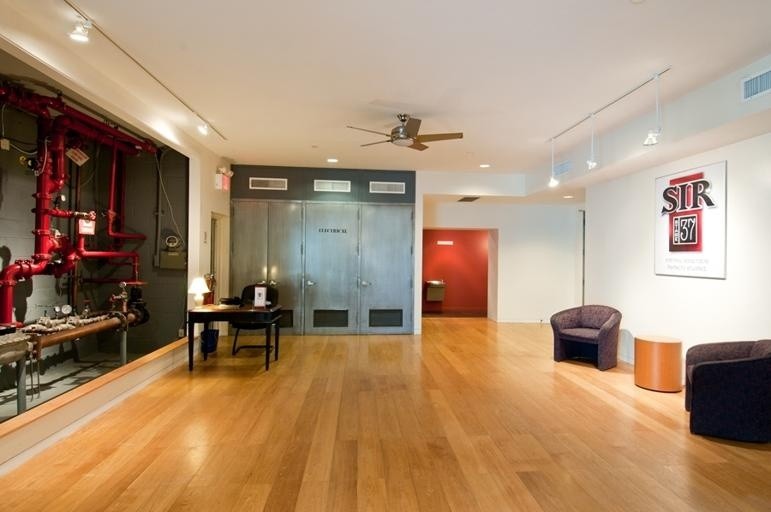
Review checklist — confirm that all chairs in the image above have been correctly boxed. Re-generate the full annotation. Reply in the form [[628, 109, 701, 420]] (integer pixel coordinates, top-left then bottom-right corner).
[[230, 284, 280, 354], [548, 304, 622, 371], [685, 339, 770, 445]]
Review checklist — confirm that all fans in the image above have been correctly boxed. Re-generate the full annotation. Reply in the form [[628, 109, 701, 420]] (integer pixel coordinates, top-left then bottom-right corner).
[[345, 113, 463, 152]]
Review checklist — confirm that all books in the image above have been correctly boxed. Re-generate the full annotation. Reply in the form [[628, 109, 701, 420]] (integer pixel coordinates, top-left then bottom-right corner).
[[218, 303, 241, 309]]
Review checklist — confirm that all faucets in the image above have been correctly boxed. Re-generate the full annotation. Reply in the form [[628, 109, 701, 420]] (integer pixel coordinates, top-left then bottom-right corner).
[[441, 279, 443, 285]]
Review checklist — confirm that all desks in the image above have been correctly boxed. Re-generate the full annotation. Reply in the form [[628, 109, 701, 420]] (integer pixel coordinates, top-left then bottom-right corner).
[[186, 303, 283, 371], [633, 336, 683, 393]]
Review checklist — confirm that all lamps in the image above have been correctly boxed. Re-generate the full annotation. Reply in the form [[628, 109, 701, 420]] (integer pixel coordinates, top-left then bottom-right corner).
[[389, 125, 416, 147], [71, 14, 212, 138], [186, 277, 210, 308], [216, 166, 234, 177], [542, 62, 674, 189]]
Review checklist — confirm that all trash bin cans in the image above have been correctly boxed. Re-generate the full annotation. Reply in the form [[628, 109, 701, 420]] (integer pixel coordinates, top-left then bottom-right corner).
[[201, 329, 219, 353]]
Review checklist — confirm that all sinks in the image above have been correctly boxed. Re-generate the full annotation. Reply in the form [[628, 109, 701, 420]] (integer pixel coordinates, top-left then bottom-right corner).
[[426, 280, 446, 285]]
